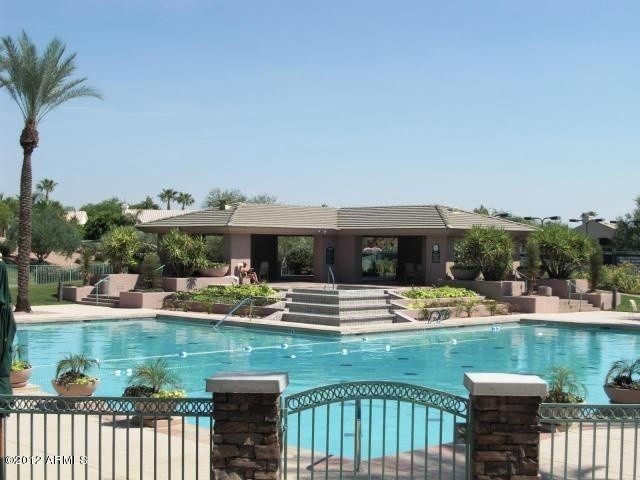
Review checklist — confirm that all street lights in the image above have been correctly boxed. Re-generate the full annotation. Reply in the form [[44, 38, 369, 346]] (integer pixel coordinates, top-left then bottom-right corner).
[[524, 216, 561, 229], [569, 218, 604, 234], [610, 220, 640, 228]]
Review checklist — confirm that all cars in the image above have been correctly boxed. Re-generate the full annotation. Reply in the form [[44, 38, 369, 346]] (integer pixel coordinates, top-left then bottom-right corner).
[[362, 247, 383, 255]]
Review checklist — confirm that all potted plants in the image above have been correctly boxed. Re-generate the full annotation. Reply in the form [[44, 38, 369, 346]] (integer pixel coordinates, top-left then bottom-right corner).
[[160, 226, 230, 276], [602, 359, 640, 404], [8, 343, 32, 383], [541, 369, 587, 430], [53, 354, 101, 397], [125, 360, 184, 418], [450, 223, 513, 280]]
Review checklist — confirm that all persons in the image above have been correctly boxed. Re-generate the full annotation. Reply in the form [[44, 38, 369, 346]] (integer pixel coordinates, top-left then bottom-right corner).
[[241, 261, 260, 285], [0, 253, 17, 480]]
